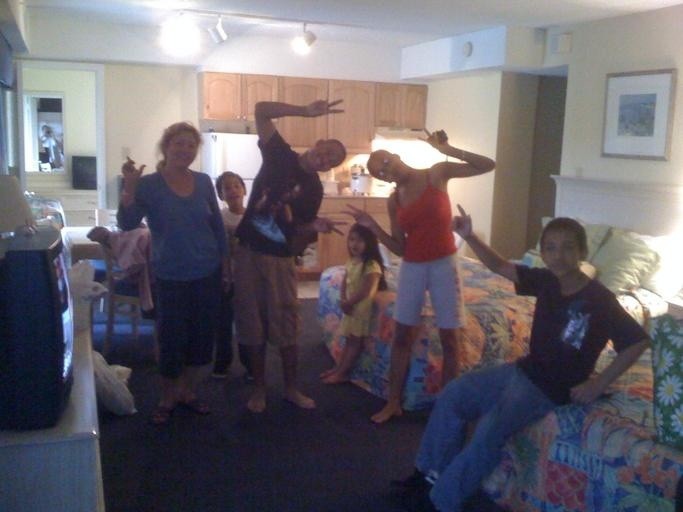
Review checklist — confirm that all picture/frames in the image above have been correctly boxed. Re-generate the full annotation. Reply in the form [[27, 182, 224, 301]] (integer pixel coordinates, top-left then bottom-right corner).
[[600, 67, 677, 160]]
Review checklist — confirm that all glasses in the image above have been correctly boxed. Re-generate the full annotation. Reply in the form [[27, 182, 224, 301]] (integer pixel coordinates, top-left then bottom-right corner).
[[377, 156, 389, 179]]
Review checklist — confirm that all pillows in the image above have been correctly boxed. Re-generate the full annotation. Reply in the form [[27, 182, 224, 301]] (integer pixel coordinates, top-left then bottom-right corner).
[[91, 349, 138, 418], [534, 216, 682, 296]]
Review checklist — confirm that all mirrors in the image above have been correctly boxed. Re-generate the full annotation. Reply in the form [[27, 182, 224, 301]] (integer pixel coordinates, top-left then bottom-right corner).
[[22, 90, 66, 175]]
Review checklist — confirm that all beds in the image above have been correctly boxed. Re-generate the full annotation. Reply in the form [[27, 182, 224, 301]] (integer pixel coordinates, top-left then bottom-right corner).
[[316, 175, 668, 411], [457, 343, 682, 511]]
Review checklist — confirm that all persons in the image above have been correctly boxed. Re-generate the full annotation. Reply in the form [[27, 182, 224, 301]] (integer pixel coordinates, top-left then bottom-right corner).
[[318, 224, 387, 383], [111, 222, 155, 319], [41, 125, 59, 168], [231, 98, 349, 412], [342, 126, 494, 423], [116, 121, 228, 425], [209, 172, 270, 381], [391, 202, 652, 512]]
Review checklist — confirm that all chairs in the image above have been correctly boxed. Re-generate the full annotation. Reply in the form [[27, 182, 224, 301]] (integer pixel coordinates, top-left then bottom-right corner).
[[101, 246, 141, 340]]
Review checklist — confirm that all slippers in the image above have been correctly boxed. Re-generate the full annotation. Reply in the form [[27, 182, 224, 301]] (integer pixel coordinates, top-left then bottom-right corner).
[[149, 402, 173, 426], [178, 397, 213, 416]]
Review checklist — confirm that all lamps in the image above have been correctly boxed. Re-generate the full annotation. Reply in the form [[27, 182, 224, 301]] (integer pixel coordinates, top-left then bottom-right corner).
[[207, 14, 227, 44], [291, 22, 317, 52]]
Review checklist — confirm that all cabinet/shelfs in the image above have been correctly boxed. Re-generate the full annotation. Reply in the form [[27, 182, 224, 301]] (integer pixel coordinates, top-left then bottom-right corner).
[[0, 272, 105, 512], [197, 71, 427, 151], [298, 198, 392, 280]]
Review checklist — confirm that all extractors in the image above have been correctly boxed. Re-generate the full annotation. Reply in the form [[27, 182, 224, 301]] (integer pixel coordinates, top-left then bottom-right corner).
[[378, 128, 426, 141]]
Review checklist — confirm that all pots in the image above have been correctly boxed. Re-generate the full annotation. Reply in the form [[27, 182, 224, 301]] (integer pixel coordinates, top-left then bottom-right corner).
[[352, 173, 373, 196]]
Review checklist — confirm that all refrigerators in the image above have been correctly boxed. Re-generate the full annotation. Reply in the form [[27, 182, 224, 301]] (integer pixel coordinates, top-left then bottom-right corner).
[[201, 131, 263, 209]]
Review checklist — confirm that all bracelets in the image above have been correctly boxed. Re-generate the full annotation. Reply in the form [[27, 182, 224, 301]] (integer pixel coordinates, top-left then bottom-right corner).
[[380, 235, 391, 243], [462, 150, 466, 162], [121, 189, 135, 198]]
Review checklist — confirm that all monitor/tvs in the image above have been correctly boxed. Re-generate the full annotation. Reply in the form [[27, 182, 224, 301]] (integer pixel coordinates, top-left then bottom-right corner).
[[0, 222, 75, 433]]
